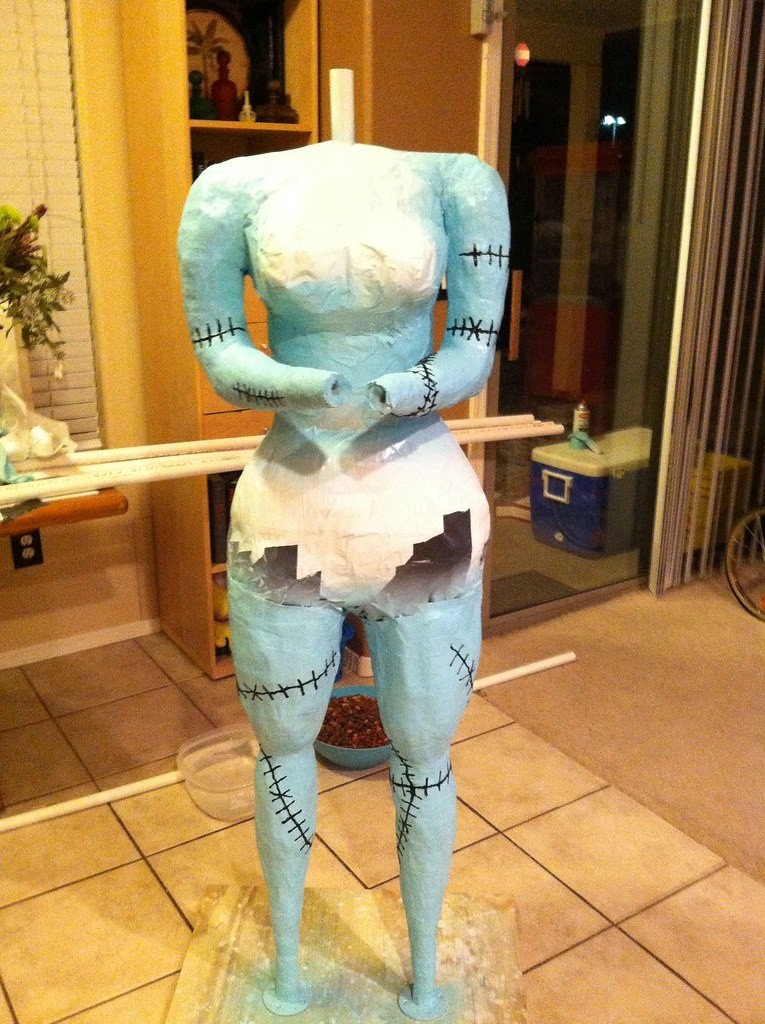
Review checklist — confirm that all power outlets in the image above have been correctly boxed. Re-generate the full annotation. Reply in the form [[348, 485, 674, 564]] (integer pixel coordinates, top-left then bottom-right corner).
[[9, 528, 44, 569]]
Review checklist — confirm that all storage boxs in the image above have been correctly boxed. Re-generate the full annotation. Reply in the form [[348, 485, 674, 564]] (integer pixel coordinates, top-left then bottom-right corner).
[[530, 426, 654, 593], [685, 453, 753, 553]]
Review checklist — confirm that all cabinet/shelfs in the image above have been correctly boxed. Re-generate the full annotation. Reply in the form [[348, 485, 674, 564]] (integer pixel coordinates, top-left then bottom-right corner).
[[120, 0, 318, 681]]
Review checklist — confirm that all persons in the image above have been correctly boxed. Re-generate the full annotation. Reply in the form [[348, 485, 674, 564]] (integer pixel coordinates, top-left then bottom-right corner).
[[176, 136, 512, 1020]]
